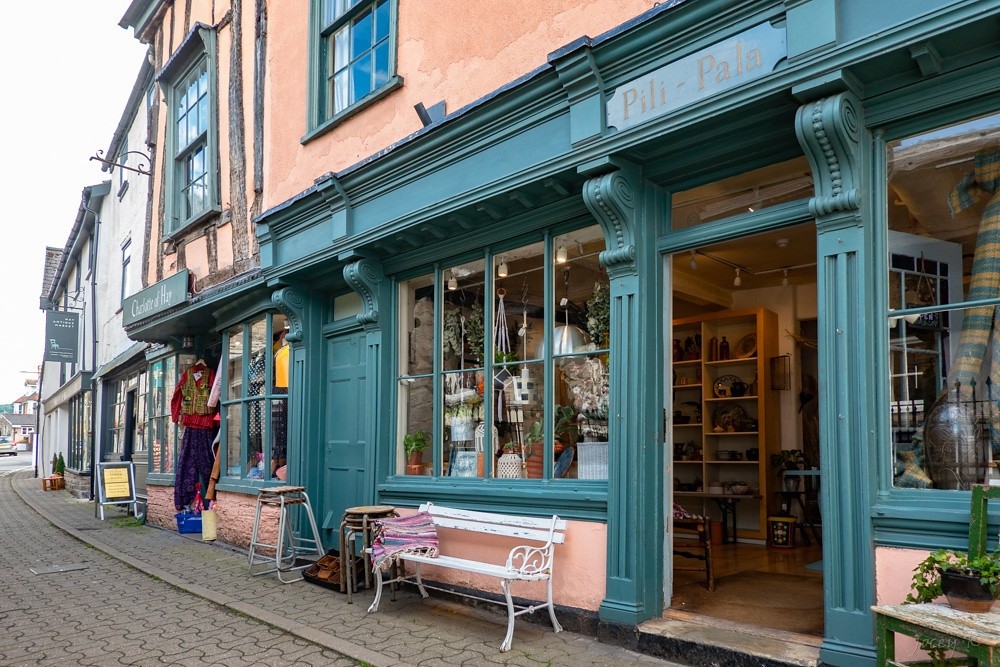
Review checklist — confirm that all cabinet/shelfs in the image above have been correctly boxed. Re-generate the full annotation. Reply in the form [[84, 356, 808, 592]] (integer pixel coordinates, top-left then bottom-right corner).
[[672, 306, 784, 546]]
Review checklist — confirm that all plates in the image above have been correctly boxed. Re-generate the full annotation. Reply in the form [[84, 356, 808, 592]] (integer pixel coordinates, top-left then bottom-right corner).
[[713, 402, 746, 432], [734, 332, 758, 358], [713, 449, 737, 460], [714, 374, 744, 397]]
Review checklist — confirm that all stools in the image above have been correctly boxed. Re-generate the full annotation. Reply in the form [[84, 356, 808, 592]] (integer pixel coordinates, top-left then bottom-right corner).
[[339, 506, 404, 604], [246, 485, 326, 583], [42, 478, 58, 491]]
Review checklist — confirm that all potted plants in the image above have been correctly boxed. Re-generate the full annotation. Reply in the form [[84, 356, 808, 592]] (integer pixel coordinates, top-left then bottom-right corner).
[[898, 548, 1000, 667], [50, 452, 65, 490], [768, 449, 816, 491], [403, 431, 433, 475], [496, 441, 524, 479], [694, 475, 748, 495], [521, 403, 577, 479], [672, 492, 763, 545]]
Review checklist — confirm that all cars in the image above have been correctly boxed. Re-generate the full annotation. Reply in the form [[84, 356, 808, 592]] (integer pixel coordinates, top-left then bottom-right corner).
[[0, 435, 17, 458]]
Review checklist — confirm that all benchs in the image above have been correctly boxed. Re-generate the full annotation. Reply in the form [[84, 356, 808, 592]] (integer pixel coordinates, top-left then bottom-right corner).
[[365, 502, 568, 654]]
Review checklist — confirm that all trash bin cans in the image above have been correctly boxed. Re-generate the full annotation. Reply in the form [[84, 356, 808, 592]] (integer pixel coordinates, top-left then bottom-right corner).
[[768, 513, 798, 548]]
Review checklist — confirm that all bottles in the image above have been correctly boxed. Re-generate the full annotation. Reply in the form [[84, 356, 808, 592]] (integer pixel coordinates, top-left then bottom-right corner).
[[709, 334, 718, 360], [720, 334, 730, 360]]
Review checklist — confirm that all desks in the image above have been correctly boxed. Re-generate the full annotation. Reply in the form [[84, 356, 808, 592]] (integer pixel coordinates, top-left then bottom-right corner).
[[771, 491, 822, 547]]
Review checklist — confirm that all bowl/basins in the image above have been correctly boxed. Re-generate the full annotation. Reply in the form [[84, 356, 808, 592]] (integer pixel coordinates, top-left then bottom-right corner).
[[734, 446, 760, 461], [708, 481, 751, 495]]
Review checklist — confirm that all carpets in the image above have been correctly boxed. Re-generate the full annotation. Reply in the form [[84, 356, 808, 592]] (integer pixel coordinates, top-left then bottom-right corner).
[[670, 570, 824, 638]]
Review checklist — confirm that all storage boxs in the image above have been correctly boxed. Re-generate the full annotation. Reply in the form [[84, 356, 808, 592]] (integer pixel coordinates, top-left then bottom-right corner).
[[301, 547, 365, 594], [175, 513, 202, 533]]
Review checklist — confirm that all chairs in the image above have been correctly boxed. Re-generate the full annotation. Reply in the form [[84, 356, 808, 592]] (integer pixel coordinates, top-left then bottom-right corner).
[[869, 484, 1000, 667]]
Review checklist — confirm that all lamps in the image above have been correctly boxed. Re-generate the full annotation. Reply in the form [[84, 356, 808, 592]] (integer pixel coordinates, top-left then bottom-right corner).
[[689, 249, 790, 287], [448, 244, 568, 290]]
